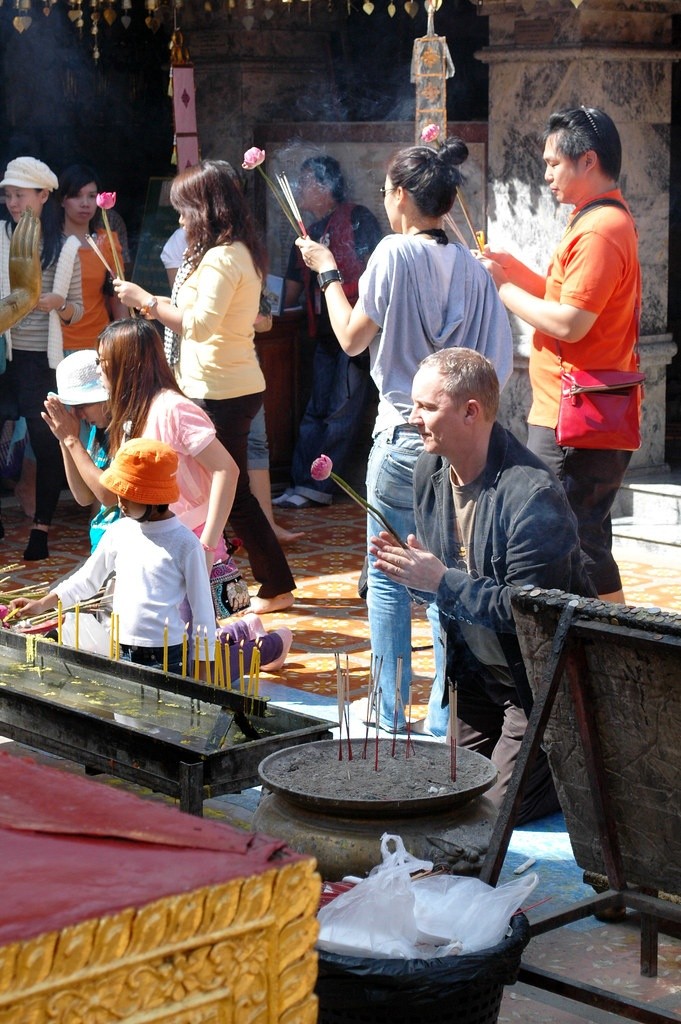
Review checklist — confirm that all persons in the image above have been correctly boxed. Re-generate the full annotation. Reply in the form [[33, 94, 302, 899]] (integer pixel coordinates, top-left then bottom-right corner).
[[468, 106, 640, 612], [294, 135, 513, 738], [40, 348, 122, 557], [369, 347, 600, 829], [95, 317, 292, 686], [10, 438, 216, 683], [272, 155, 385, 508], [0, 159, 298, 615]]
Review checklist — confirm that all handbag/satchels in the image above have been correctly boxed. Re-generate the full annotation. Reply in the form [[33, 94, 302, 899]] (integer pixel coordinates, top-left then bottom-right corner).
[[315, 832, 539, 959], [557, 369, 643, 451]]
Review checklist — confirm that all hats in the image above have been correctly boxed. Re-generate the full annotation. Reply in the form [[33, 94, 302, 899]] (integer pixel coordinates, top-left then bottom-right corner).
[[48, 349, 112, 405], [0, 156, 59, 192], [99, 438, 181, 504]]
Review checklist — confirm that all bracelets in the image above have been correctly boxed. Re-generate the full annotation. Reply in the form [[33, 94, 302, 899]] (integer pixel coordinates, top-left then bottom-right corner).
[[139, 297, 157, 317], [58, 303, 66, 311], [317, 270, 344, 291], [202, 544, 216, 552]]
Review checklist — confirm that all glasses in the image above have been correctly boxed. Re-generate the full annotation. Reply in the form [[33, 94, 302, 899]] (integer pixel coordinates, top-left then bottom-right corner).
[[380, 187, 396, 197]]
